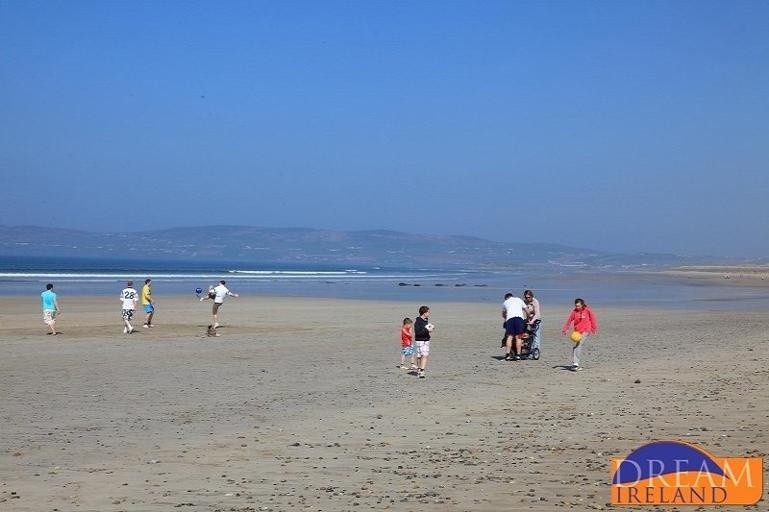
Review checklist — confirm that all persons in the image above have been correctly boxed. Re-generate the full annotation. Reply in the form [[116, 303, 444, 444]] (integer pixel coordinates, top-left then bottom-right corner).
[[501, 289, 542, 362], [40, 283, 61, 336], [561, 298, 597, 370], [199, 280, 239, 329], [399, 318, 418, 370], [141, 279, 155, 329], [413, 305, 433, 379], [119, 280, 139, 334]]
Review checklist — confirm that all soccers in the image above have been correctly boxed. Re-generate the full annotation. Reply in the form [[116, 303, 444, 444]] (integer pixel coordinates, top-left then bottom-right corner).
[[426, 324, 434, 332], [571, 330, 582, 342], [196, 287, 202, 294]]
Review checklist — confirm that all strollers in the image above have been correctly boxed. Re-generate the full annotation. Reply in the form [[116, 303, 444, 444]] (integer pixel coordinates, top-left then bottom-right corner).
[[503, 319, 541, 359]]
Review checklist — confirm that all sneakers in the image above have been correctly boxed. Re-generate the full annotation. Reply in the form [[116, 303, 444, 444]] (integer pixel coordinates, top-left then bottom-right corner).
[[123, 326, 134, 334], [571, 365, 582, 372], [516, 356, 522, 362], [501, 357, 514, 361], [142, 324, 153, 329], [400, 363, 425, 378]]
